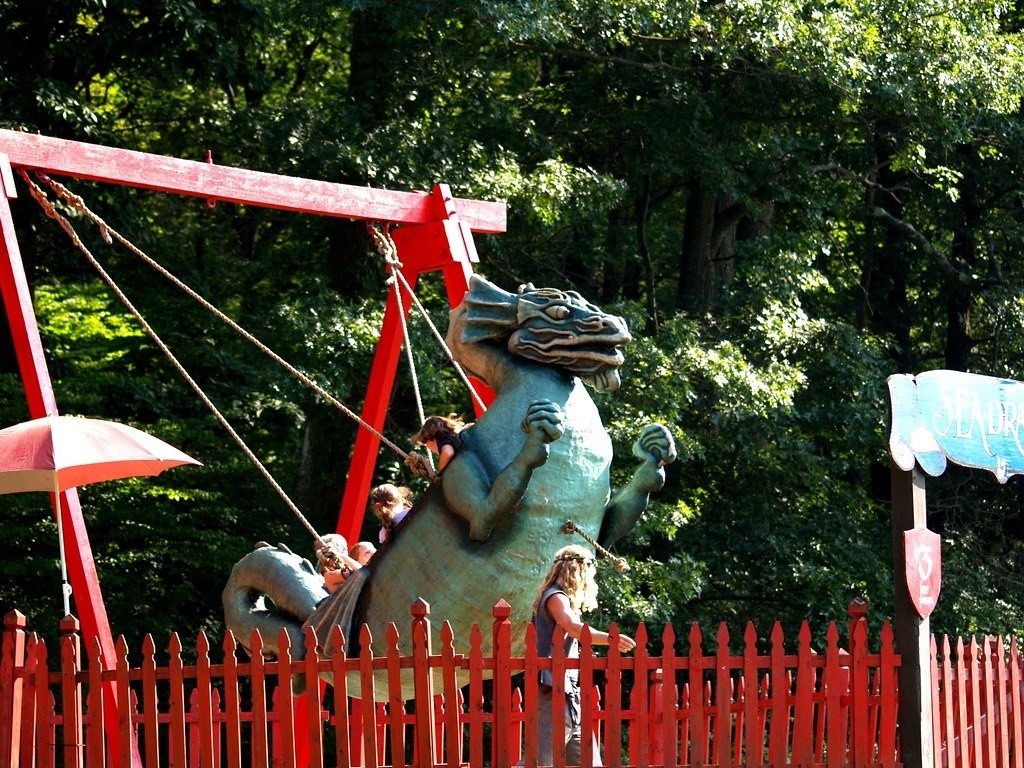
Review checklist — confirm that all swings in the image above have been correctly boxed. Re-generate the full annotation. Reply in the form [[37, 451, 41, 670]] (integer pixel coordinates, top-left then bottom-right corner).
[[29, 170, 635, 707]]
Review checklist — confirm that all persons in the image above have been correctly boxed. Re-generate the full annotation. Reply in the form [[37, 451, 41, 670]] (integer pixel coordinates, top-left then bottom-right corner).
[[518, 544, 637, 767], [409, 412, 474, 472], [315, 483, 415, 595]]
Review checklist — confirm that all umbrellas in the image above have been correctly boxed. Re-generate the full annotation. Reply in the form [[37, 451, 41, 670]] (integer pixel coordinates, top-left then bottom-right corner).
[[0, 413, 205, 616]]
